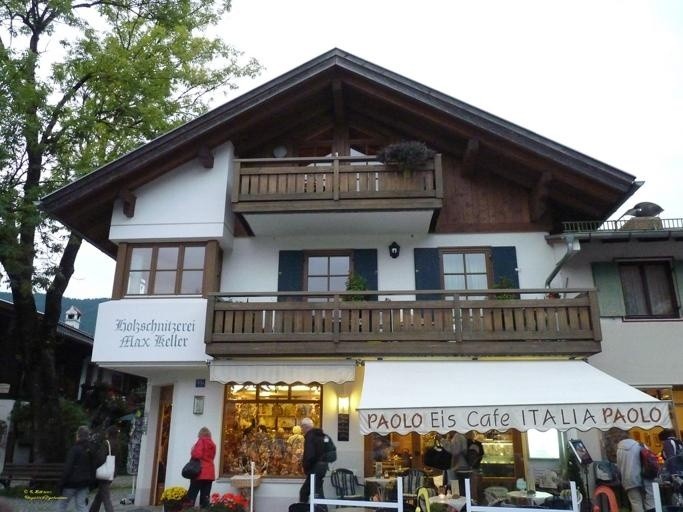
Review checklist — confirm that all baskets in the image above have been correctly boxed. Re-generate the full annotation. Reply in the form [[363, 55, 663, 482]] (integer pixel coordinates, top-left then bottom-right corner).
[[231, 475, 260, 488]]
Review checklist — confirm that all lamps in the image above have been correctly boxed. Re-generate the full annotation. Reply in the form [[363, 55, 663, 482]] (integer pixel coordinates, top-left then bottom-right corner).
[[389, 241, 400, 259]]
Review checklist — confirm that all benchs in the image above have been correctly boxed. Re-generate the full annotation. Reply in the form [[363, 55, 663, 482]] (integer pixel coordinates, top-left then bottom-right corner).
[[0, 462, 66, 497]]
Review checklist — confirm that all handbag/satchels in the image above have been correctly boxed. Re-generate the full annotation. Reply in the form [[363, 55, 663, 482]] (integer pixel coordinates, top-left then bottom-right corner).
[[424, 447, 451, 470], [182, 460, 200, 479], [640, 446, 659, 479], [671, 493, 683, 507], [95, 455, 116, 482], [314, 430, 337, 463]]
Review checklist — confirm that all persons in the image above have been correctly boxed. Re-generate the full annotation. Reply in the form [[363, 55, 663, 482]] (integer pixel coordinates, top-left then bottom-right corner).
[[605, 427, 623, 464], [434, 430, 469, 512], [49, 425, 99, 512], [464, 430, 485, 506], [299, 416, 330, 512], [89, 426, 128, 512], [613, 429, 656, 512], [185, 425, 217, 510], [658, 429, 683, 512], [241, 418, 305, 475]]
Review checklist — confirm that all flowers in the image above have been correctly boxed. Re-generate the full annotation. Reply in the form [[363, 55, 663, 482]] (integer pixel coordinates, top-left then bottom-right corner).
[[160, 486, 248, 512]]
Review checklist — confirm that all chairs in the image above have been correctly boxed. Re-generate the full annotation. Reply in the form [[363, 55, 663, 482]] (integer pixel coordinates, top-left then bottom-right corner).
[[483, 485, 584, 512], [329, 467, 429, 512]]
